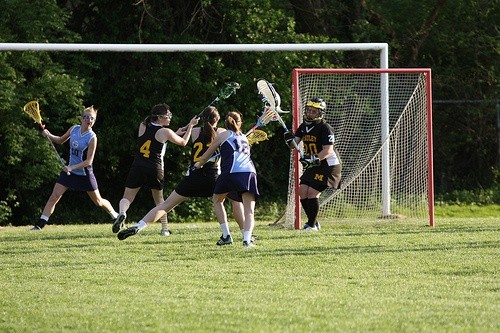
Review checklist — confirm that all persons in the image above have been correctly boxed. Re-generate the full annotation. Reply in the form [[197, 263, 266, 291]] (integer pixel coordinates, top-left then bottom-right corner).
[[118, 103, 257, 241], [284, 97, 343, 231], [112, 104, 173, 236], [29, 105, 128, 230], [194, 111, 259, 246]]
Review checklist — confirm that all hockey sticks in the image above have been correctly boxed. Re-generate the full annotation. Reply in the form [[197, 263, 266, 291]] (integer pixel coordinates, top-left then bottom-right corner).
[[257, 79, 304, 159], [23, 101, 70, 176], [184, 82, 240, 129], [191, 130, 267, 172], [245, 108, 278, 137]]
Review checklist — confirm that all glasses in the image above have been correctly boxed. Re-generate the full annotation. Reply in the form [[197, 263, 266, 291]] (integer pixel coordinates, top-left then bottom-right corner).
[[82, 114, 96, 124], [159, 112, 173, 119]]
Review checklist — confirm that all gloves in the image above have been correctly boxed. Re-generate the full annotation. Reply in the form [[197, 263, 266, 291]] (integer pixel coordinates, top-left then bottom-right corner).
[[299, 154, 320, 169], [284, 131, 295, 145], [35, 120, 46, 132]]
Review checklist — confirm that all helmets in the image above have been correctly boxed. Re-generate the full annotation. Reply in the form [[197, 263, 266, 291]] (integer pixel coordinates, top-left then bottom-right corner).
[[303, 97, 327, 124]]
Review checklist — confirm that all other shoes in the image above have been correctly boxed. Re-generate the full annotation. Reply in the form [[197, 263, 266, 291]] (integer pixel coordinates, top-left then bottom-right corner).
[[112, 218, 124, 227], [30, 225, 41, 231]]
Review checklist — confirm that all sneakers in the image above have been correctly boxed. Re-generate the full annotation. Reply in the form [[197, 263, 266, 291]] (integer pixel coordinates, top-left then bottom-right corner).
[[112, 212, 126, 233], [250, 236, 255, 242], [217, 234, 233, 245], [303, 224, 318, 230], [316, 222, 321, 230], [243, 241, 256, 247], [161, 229, 172, 236], [117, 227, 139, 240]]
